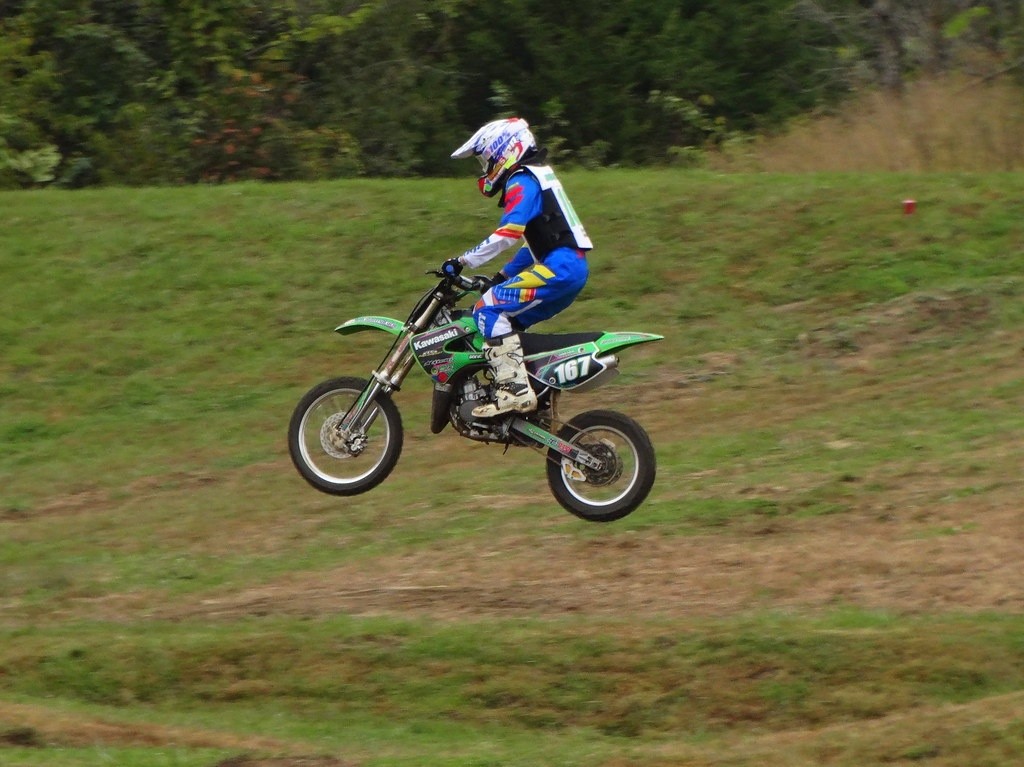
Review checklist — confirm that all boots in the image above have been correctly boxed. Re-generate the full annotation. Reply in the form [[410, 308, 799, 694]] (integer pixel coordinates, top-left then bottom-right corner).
[[470, 330, 538, 417]]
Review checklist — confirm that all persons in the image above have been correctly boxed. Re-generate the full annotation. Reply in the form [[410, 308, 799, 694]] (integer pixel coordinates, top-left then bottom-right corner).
[[441, 118, 589, 420]]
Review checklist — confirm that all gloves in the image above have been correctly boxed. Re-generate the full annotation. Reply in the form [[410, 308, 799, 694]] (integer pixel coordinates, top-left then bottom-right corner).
[[480, 272, 507, 295], [441, 257, 463, 281]]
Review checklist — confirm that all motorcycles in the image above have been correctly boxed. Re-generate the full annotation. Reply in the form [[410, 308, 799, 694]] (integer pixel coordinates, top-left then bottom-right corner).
[[285, 260, 666, 523]]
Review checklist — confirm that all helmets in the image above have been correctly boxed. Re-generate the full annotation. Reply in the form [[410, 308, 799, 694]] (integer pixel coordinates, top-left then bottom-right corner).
[[451, 117, 540, 198]]
